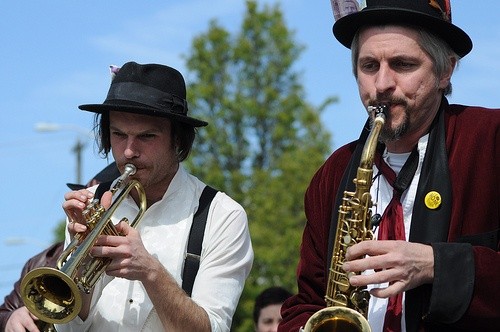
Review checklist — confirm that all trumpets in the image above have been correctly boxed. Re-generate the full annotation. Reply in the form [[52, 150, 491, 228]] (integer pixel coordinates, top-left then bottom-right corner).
[[19, 163, 147, 324]]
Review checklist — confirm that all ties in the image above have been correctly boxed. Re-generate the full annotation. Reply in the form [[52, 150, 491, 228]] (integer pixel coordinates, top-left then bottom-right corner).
[[374, 144, 419, 332]]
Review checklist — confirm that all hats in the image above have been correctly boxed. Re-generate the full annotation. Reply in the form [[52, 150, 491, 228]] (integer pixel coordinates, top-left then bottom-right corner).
[[333, 0, 472, 58], [79, 62, 209, 127]]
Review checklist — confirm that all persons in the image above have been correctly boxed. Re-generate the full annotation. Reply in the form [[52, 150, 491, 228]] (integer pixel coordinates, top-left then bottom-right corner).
[[0, 161, 122, 332], [54, 61, 254, 332], [252, 287, 292, 332], [278, 0, 500, 332]]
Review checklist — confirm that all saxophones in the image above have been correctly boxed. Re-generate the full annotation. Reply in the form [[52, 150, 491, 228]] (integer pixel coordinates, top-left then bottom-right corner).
[[303, 102, 389, 332]]
